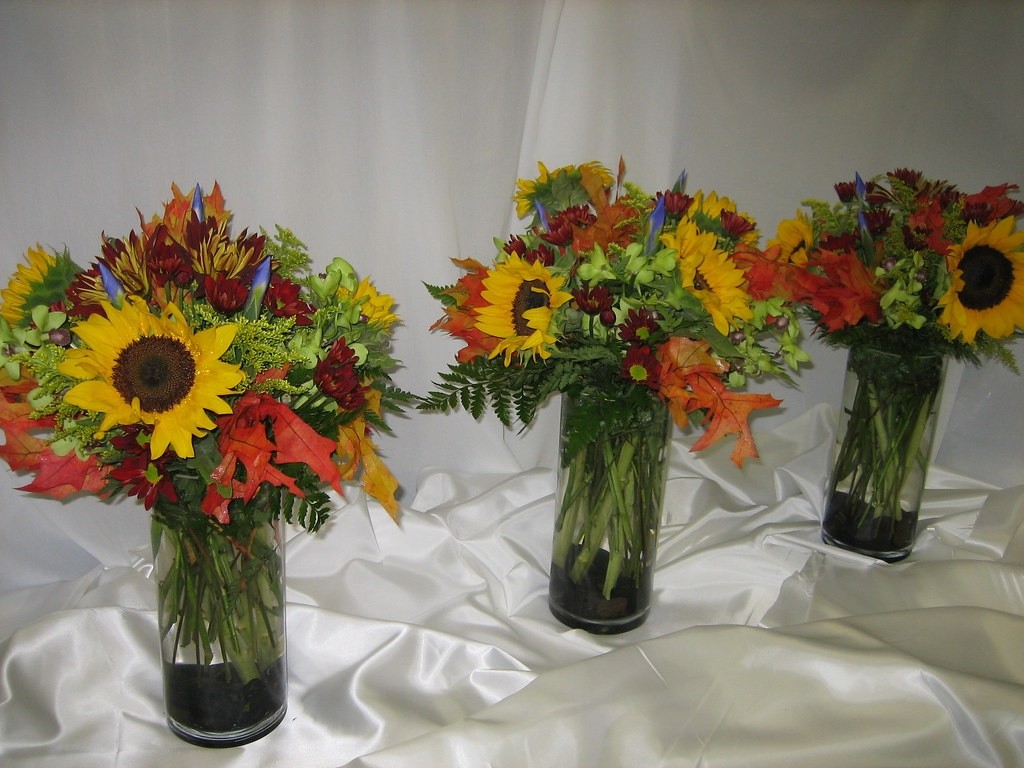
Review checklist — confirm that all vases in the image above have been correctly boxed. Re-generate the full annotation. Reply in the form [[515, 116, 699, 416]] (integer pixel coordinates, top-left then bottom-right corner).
[[548, 381, 674, 635], [150, 487, 288, 748], [821, 345, 950, 563]]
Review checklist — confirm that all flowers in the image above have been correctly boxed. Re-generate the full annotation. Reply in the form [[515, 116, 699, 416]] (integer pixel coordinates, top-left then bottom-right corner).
[[416, 155, 1024, 601], [1, 181, 433, 684]]
